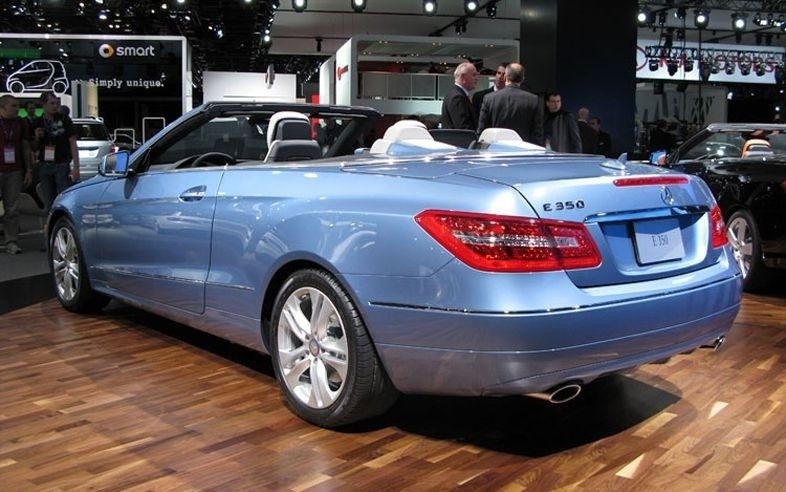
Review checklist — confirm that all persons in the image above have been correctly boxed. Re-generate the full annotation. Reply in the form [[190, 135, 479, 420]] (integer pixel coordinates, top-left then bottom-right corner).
[[28, 92, 80, 230], [317, 117, 349, 149], [58, 105, 69, 118], [441, 61, 611, 161], [648, 119, 675, 151], [22, 99, 39, 133], [0, 94, 33, 256]]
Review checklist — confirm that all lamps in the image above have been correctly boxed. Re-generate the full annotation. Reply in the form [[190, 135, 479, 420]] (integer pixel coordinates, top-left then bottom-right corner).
[[644, 45, 785, 76], [259, 0, 498, 57], [634, 2, 786, 34]]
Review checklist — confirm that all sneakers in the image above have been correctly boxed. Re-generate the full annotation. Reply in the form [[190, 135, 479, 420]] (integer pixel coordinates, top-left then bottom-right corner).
[[40, 238, 46, 249], [6, 242, 22, 255]]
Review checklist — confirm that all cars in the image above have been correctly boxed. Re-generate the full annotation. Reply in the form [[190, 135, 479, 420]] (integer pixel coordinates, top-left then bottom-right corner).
[[675, 141, 740, 157]]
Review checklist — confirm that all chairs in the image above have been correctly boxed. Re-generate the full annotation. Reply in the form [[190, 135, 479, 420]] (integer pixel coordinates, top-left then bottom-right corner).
[[739, 138, 773, 161], [258, 102, 333, 167], [368, 114, 441, 155], [473, 125, 528, 149]]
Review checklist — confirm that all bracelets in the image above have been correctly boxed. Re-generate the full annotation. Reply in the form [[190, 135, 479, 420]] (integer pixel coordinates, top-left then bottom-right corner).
[[26, 169, 33, 172]]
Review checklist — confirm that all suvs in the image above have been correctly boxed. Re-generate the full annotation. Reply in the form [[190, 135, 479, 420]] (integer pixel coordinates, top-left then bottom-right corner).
[[67, 112, 119, 181]]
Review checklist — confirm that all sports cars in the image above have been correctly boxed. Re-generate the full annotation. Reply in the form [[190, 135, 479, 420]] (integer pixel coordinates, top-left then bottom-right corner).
[[655, 122, 786, 291], [41, 98, 748, 432]]
